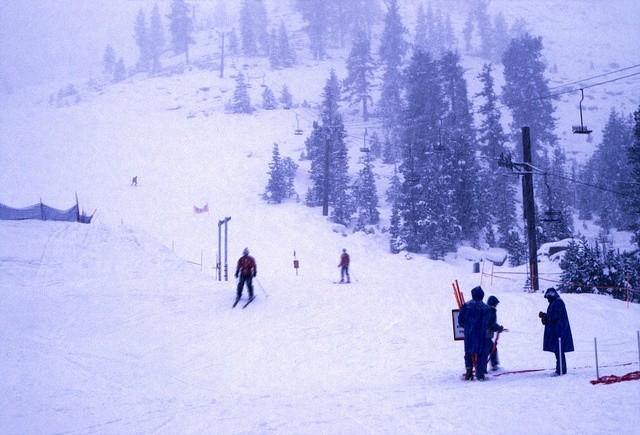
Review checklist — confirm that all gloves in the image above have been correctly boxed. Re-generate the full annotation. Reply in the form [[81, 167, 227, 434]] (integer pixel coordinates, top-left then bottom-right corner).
[[539, 311, 544, 317], [541, 317, 547, 325]]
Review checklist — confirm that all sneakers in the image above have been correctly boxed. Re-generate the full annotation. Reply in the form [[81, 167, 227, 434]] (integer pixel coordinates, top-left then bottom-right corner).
[[549, 373, 563, 377], [490, 367, 503, 372], [477, 376, 489, 381], [466, 373, 474, 380]]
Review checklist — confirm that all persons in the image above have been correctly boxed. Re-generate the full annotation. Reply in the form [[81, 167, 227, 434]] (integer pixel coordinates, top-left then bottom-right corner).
[[537, 287, 575, 376], [458, 285, 492, 381], [486, 295, 509, 372], [336, 249, 352, 283], [233, 248, 258, 303]]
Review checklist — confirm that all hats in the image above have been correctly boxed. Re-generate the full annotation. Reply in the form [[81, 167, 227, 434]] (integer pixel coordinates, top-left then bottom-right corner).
[[242, 248, 249, 254]]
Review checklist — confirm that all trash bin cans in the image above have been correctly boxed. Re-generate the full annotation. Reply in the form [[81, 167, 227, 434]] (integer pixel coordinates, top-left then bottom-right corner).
[[473, 262, 480, 273]]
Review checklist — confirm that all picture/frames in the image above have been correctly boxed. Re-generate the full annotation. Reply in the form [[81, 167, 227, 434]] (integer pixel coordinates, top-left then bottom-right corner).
[[452, 309, 465, 340]]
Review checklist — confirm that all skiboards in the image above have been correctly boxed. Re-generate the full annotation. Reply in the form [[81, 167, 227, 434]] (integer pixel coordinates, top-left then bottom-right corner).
[[233, 293, 256, 309]]
